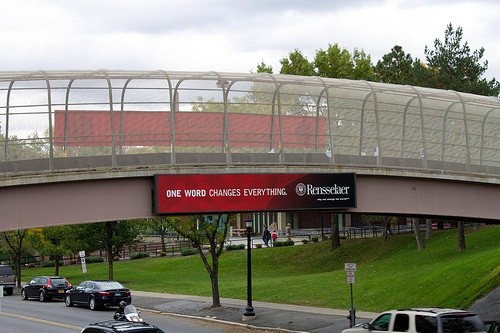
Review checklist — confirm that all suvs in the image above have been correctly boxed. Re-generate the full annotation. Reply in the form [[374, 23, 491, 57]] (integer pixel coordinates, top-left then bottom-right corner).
[[342, 306, 494, 333]]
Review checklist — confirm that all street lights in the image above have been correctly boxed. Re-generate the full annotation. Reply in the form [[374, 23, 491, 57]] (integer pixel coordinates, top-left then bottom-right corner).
[[243, 216, 255, 316]]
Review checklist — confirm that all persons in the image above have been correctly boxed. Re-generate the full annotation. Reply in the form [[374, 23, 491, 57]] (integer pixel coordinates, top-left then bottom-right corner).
[[270, 220, 278, 229], [262, 226, 271, 247], [271, 230, 278, 243], [116, 301, 128, 320], [286, 222, 291, 240]]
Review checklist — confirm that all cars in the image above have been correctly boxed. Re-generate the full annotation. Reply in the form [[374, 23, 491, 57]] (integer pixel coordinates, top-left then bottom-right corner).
[[20, 276, 73, 302], [65, 279, 132, 310], [80, 319, 166, 333]]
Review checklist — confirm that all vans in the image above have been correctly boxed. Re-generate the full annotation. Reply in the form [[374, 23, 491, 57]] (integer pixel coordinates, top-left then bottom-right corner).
[[0, 264, 16, 295]]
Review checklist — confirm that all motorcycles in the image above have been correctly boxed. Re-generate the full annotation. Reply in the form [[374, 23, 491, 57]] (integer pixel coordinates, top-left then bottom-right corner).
[[113, 305, 144, 322]]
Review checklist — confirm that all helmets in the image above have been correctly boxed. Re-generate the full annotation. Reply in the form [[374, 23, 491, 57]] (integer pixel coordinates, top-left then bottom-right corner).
[[120, 300, 127, 306]]
